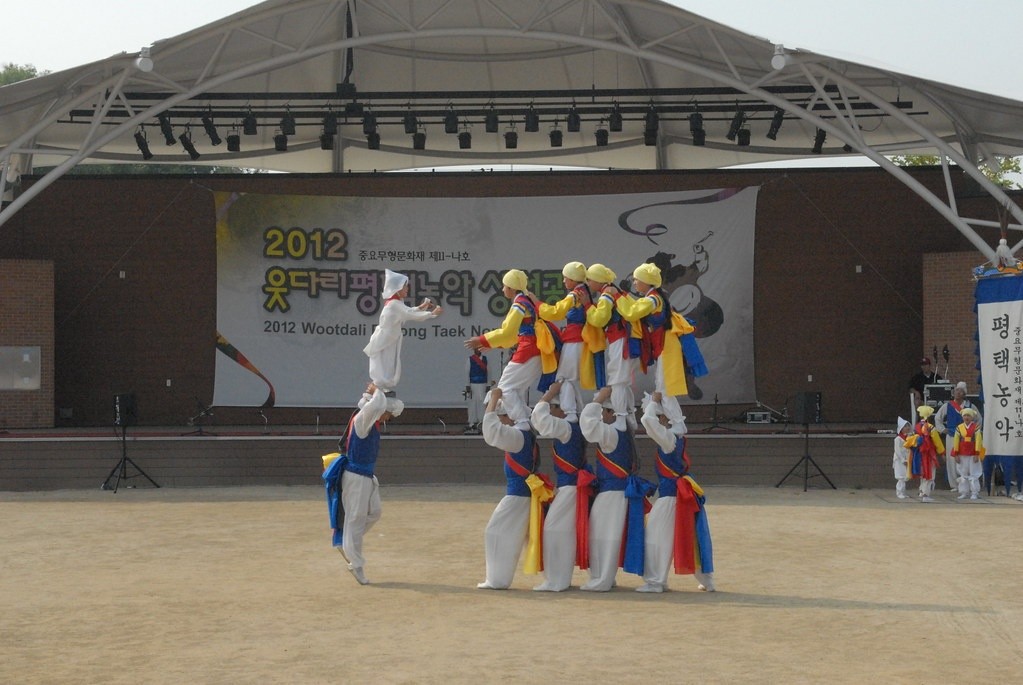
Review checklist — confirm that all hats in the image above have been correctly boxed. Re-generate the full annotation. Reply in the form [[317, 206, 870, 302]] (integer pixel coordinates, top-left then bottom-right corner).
[[502, 269, 529, 296], [916, 405, 934, 421], [920, 358, 930, 364], [897, 416, 911, 434], [633, 262, 663, 289], [960, 408, 977, 419], [956, 381, 967, 395], [382, 268, 409, 299], [586, 263, 617, 285], [563, 262, 586, 282], [483, 391, 665, 416]]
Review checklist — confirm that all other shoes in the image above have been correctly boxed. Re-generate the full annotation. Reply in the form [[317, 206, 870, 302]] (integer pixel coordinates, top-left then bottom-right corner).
[[635, 584, 668, 593], [950, 489, 958, 492], [918, 492, 923, 498], [922, 496, 935, 503], [957, 494, 969, 499], [532, 581, 571, 592], [698, 583, 715, 592], [897, 494, 912, 499], [970, 495, 978, 499], [477, 582, 491, 588], [580, 577, 617, 591]]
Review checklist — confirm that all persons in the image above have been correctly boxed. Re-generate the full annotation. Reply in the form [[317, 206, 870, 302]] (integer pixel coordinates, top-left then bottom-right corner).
[[893, 415, 911, 498], [323, 261, 716, 592], [934, 381, 983, 492], [910, 358, 942, 425], [950, 408, 983, 500], [904, 406, 946, 503]]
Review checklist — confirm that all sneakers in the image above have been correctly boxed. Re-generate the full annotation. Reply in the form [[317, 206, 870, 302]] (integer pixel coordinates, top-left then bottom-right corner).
[[337, 545, 369, 585]]
